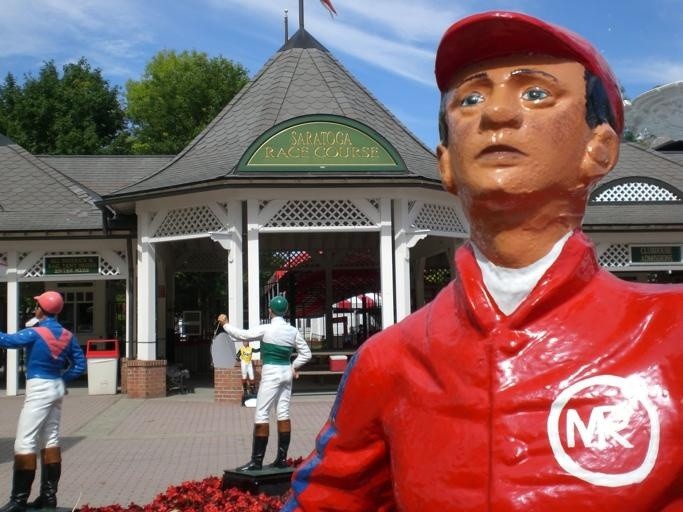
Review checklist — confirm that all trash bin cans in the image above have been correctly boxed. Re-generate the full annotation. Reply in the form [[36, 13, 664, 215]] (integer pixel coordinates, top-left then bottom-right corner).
[[85, 339, 120, 395]]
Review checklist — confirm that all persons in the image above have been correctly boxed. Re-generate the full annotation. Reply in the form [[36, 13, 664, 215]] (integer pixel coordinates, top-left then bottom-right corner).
[[216, 295, 313, 473], [0, 289, 86, 511], [233, 339, 261, 400], [272, 8, 683, 511]]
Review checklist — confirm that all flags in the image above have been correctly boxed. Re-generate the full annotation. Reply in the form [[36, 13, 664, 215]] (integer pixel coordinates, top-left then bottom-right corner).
[[318, 0, 339, 22]]
